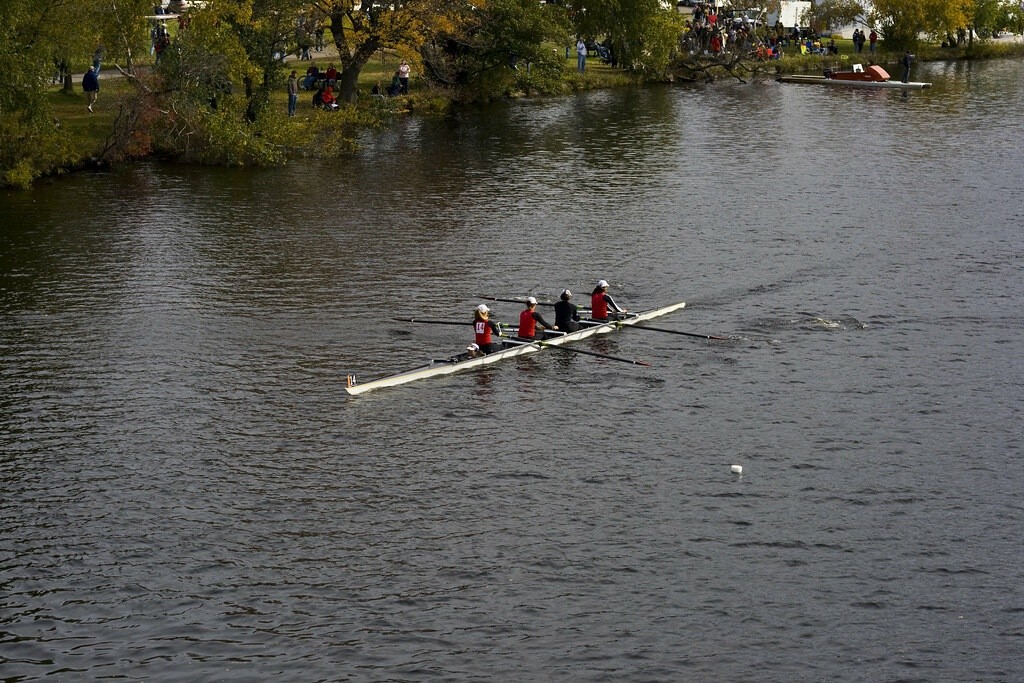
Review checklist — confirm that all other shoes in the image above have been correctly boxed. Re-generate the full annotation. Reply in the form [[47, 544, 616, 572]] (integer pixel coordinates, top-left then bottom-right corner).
[[87, 106, 93, 113]]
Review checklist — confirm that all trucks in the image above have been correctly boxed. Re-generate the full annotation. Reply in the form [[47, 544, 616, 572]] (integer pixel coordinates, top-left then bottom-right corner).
[[732, 0, 811, 38]]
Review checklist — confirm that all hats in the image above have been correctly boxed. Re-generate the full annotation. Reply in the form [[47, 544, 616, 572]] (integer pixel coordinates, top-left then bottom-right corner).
[[476, 304, 490, 313], [466, 343, 480, 350], [526, 296, 538, 304], [560, 289, 572, 297], [597, 279, 610, 288]]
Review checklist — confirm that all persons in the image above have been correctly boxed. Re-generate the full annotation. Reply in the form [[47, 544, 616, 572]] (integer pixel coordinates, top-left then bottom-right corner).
[[901, 50, 911, 83], [472, 304, 504, 353], [822, 66, 837, 78], [300, 61, 319, 90], [82, 56, 101, 113], [313, 86, 336, 107], [584, 36, 626, 69], [518, 296, 558, 340], [808, 29, 838, 54], [682, 3, 800, 62], [853, 29, 877, 52], [52, 57, 65, 83], [150, 4, 184, 65], [323, 63, 336, 87], [301, 23, 324, 60], [577, 39, 587, 73], [287, 70, 298, 115], [372, 60, 411, 97], [942, 38, 957, 48], [591, 280, 627, 320], [553, 289, 582, 333]]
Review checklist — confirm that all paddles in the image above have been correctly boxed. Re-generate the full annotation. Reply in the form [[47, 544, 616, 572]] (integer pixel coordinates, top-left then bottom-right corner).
[[479, 297, 592, 310], [490, 332, 656, 367], [580, 316, 734, 341], [389, 317, 541, 330]]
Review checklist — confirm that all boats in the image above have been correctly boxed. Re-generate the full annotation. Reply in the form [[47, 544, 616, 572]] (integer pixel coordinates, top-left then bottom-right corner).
[[780, 65, 933, 90], [344, 301, 685, 396]]
